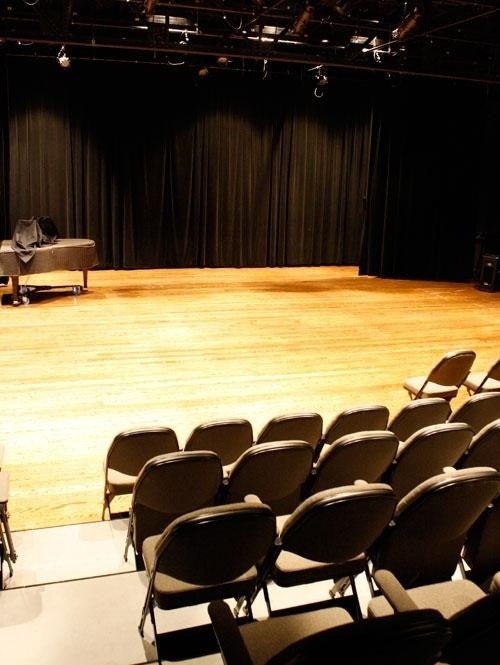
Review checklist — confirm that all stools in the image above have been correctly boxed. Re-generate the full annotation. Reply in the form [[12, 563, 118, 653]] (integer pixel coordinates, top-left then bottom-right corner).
[[1, 472, 26, 583]]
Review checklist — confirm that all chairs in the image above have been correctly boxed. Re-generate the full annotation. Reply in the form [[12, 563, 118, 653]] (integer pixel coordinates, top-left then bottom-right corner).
[[305, 430, 400, 510], [387, 397, 451, 443], [490, 570, 499, 593], [255, 411, 324, 460], [365, 567, 499, 665], [121, 447, 224, 564], [220, 438, 314, 517], [378, 423, 474, 502], [323, 404, 390, 452], [402, 347, 478, 421], [100, 426, 182, 523], [455, 418, 500, 471], [458, 506, 499, 582], [448, 392, 500, 434], [461, 355, 500, 398], [205, 596, 445, 665], [367, 464, 500, 594], [136, 493, 278, 665], [244, 478, 395, 617], [185, 419, 254, 483]]
